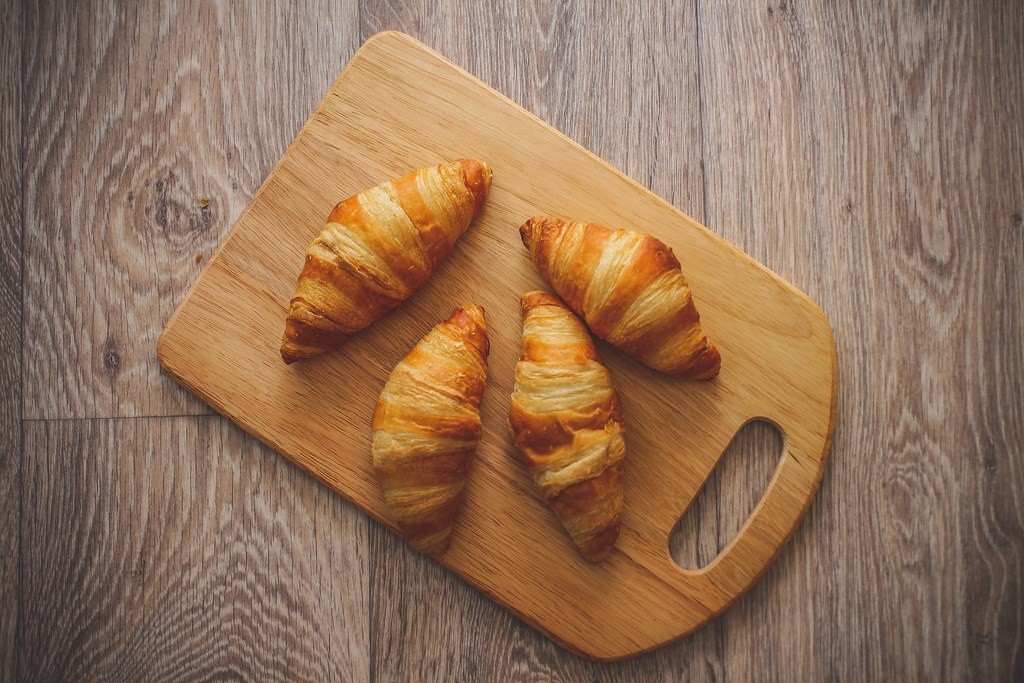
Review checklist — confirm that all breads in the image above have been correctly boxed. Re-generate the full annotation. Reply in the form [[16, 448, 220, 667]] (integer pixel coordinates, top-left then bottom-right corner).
[[280, 158, 494, 362], [520, 219, 721, 381], [511, 291, 626, 562], [369, 304, 490, 559]]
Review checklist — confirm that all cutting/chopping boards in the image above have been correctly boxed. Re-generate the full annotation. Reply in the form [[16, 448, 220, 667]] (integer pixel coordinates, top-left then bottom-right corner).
[[156, 31, 839, 660]]
